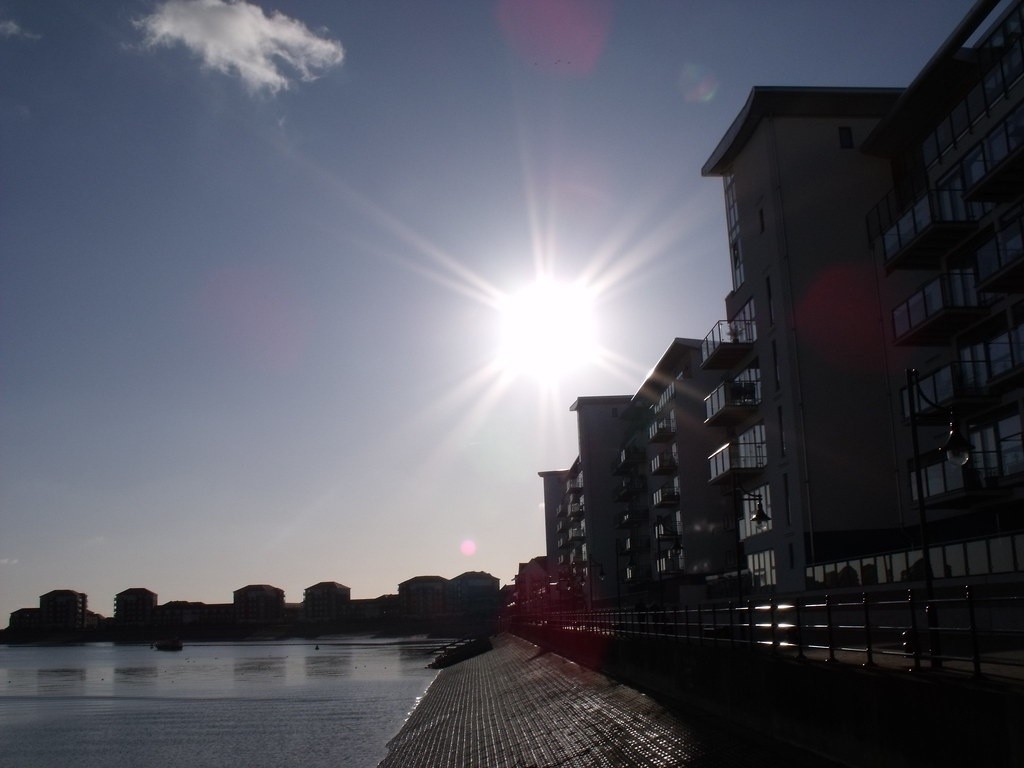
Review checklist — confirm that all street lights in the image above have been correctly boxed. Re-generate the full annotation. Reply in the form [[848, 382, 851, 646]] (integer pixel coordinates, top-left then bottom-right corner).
[[588, 554, 607, 621], [905, 365, 976, 670], [656, 514, 684, 636], [615, 538, 633, 637], [558, 571, 572, 627], [731, 467, 771, 650]]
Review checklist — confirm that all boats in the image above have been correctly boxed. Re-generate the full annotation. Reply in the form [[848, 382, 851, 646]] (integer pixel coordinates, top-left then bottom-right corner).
[[155, 639, 183, 652]]
